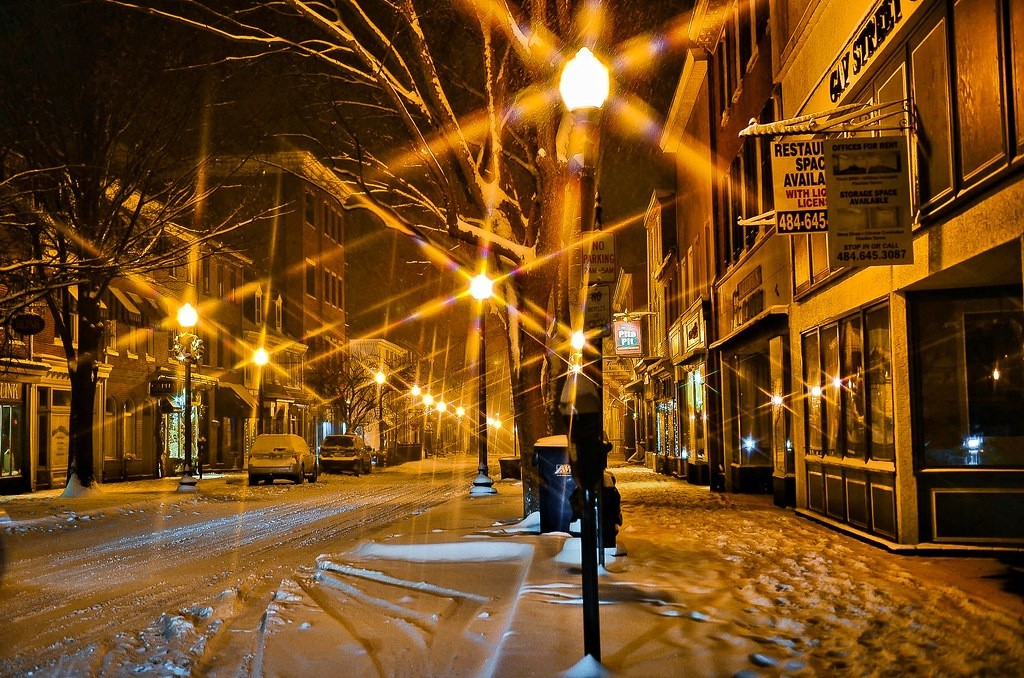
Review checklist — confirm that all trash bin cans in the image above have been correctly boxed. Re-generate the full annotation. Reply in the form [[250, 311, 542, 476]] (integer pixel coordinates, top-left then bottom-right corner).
[[531, 435, 580, 538], [498, 456, 521, 481]]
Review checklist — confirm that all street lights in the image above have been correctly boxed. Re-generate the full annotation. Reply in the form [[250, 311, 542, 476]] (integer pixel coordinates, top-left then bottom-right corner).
[[469, 273, 500, 499], [253, 348, 270, 437], [375, 370, 390, 467], [412, 386, 465, 458], [552, 47, 620, 677], [176, 303, 200, 493]]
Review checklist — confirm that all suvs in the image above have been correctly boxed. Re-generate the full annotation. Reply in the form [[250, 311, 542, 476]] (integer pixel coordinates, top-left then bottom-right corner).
[[248, 431, 318, 485], [317, 434, 373, 476]]
